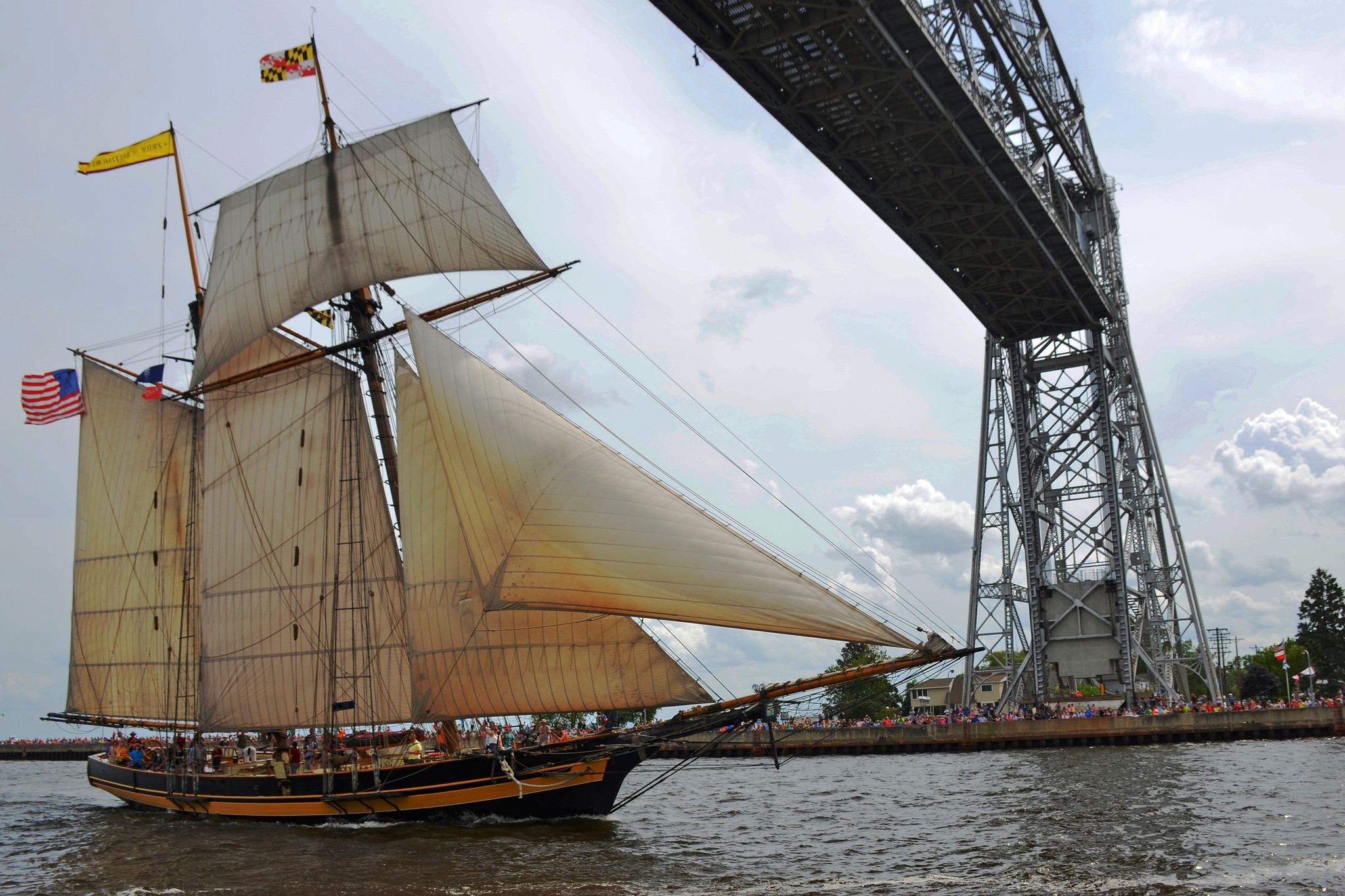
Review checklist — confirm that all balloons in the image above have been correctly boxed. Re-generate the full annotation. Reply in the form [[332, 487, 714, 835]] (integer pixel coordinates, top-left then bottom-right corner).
[[604, 716, 608, 721]]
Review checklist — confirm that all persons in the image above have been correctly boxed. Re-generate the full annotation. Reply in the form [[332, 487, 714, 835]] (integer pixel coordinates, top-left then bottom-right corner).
[[1070, 689, 1073, 697], [1055, 683, 1059, 694], [0, 713, 606, 774], [1076, 691, 1081, 697], [1022, 701, 1136, 720], [641, 707, 946, 733], [944, 702, 1024, 724], [1134, 688, 1345, 717]]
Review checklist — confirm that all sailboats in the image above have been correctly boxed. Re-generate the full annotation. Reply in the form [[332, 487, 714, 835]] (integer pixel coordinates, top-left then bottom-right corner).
[[39, 31, 987, 826]]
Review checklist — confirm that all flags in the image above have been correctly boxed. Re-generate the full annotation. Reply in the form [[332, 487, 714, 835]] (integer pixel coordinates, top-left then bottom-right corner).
[[1299, 666, 1315, 677], [77, 129, 173, 175], [1282, 659, 1290, 670], [134, 363, 164, 385], [1292, 675, 1300, 681], [142, 383, 162, 399], [1272, 642, 1283, 652], [304, 307, 333, 329], [260, 43, 316, 84], [1274, 649, 1285, 661], [21, 368, 86, 424]]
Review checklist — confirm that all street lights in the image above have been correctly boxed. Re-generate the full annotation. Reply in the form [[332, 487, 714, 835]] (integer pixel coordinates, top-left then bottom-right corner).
[[1302, 650, 1316, 707]]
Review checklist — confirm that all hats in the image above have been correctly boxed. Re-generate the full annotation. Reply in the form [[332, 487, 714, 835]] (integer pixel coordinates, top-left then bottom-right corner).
[[505, 726, 510, 730], [130, 732, 137, 736], [222, 735, 227, 738], [111, 740, 120, 746], [539, 719, 547, 723], [134, 744, 141, 749]]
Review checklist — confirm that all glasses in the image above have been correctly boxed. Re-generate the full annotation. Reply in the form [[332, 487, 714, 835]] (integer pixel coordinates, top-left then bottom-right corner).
[[493, 728, 497, 730]]
